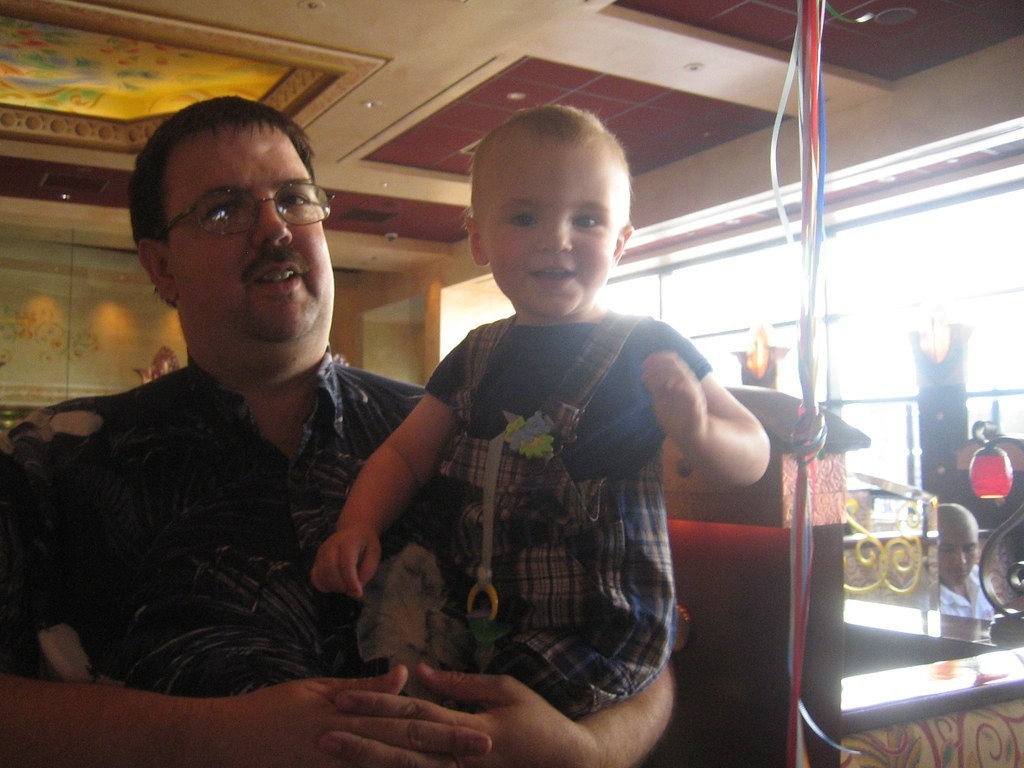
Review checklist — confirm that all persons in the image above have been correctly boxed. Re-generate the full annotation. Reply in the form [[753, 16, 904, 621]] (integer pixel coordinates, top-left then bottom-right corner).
[[0, 97, 673, 768], [897, 502, 999, 620], [316, 105, 770, 717]]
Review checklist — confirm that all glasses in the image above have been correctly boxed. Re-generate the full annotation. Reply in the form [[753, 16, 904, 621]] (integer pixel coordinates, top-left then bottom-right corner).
[[156, 182, 335, 241]]
